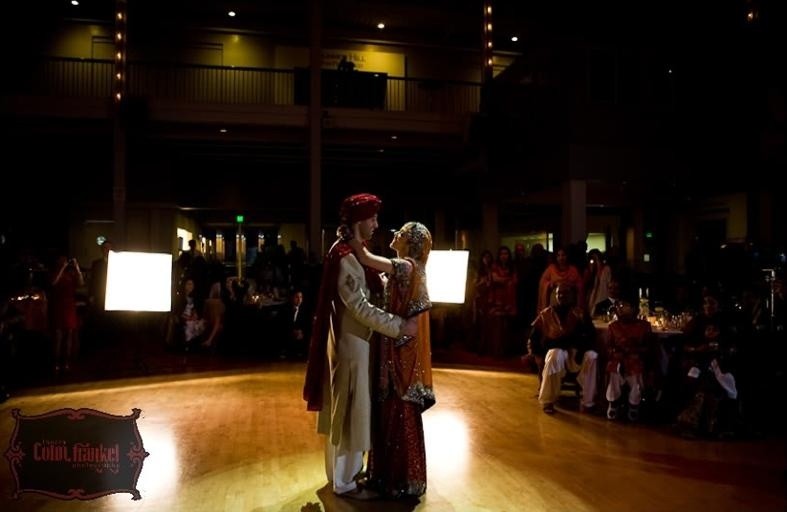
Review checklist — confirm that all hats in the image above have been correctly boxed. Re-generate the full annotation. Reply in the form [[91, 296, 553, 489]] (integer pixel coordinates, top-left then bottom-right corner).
[[340, 192, 382, 222]]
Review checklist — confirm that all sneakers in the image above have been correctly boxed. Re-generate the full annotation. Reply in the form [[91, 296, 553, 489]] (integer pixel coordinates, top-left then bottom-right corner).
[[606, 401, 618, 420], [544, 402, 555, 415], [627, 404, 640, 422], [579, 398, 605, 416]]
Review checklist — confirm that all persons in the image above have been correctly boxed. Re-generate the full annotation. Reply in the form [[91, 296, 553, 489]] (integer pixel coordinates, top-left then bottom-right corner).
[[334, 219, 433, 508], [2, 235, 619, 380], [523, 250, 787, 444], [301, 191, 420, 503]]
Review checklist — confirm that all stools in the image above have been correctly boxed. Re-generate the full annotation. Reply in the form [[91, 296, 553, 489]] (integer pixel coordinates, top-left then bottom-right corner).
[[538, 368, 580, 409]]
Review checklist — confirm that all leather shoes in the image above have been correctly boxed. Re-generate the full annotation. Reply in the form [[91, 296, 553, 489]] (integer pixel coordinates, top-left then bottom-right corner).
[[341, 486, 379, 500]]
